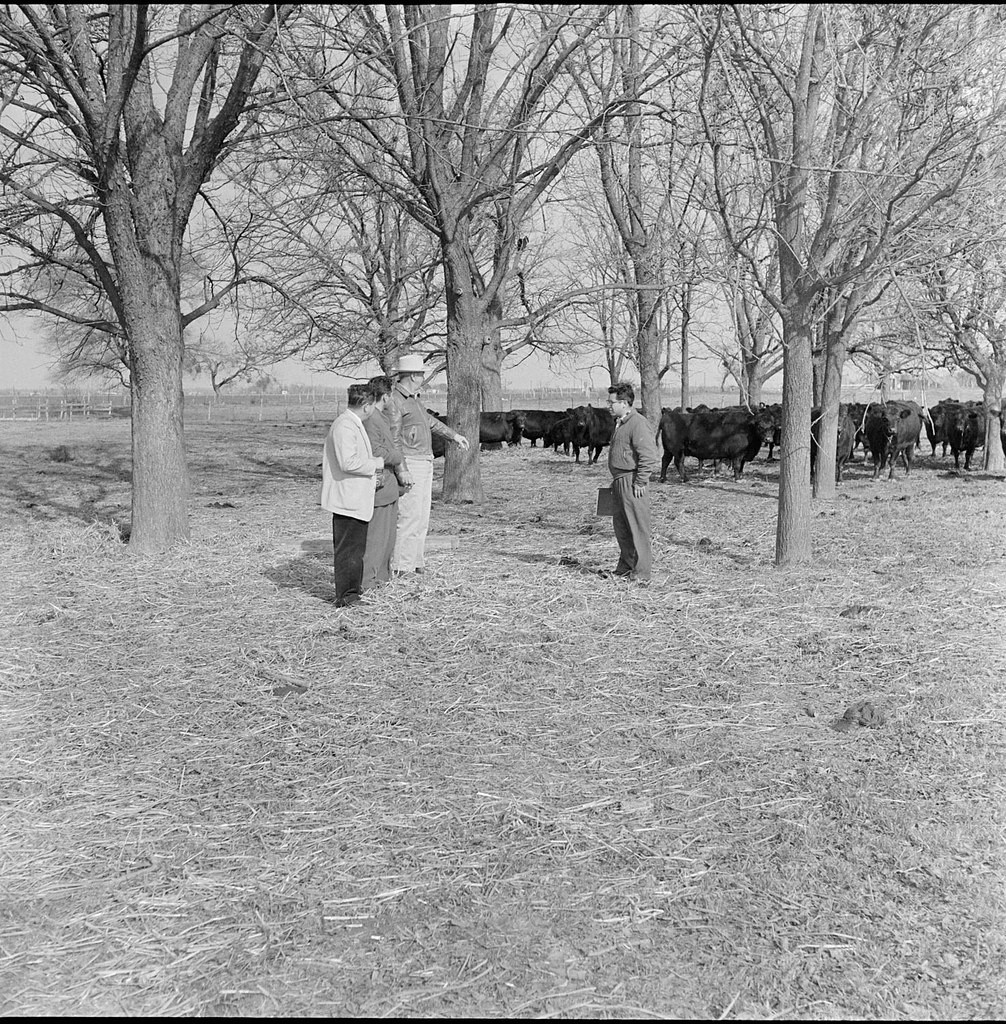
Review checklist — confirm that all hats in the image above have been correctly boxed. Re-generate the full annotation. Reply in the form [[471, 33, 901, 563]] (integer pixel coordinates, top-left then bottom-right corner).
[[392, 354, 431, 372]]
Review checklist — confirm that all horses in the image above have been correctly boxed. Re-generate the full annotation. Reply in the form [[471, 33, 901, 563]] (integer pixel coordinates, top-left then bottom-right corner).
[[426, 398, 1006, 484]]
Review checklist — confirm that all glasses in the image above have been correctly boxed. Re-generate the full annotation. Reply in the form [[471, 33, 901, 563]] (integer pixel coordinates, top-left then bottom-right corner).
[[606, 400, 622, 406]]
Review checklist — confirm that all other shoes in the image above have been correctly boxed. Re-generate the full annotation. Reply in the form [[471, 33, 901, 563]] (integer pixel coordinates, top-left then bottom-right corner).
[[416, 568, 425, 573], [337, 599, 371, 608], [627, 579, 649, 589], [394, 570, 416, 580], [602, 571, 629, 579]]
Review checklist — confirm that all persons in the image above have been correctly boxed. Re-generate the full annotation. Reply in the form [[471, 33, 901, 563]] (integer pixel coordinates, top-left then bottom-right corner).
[[362, 376, 414, 593], [382, 355, 470, 579], [320, 384, 384, 609], [598, 383, 657, 589]]
